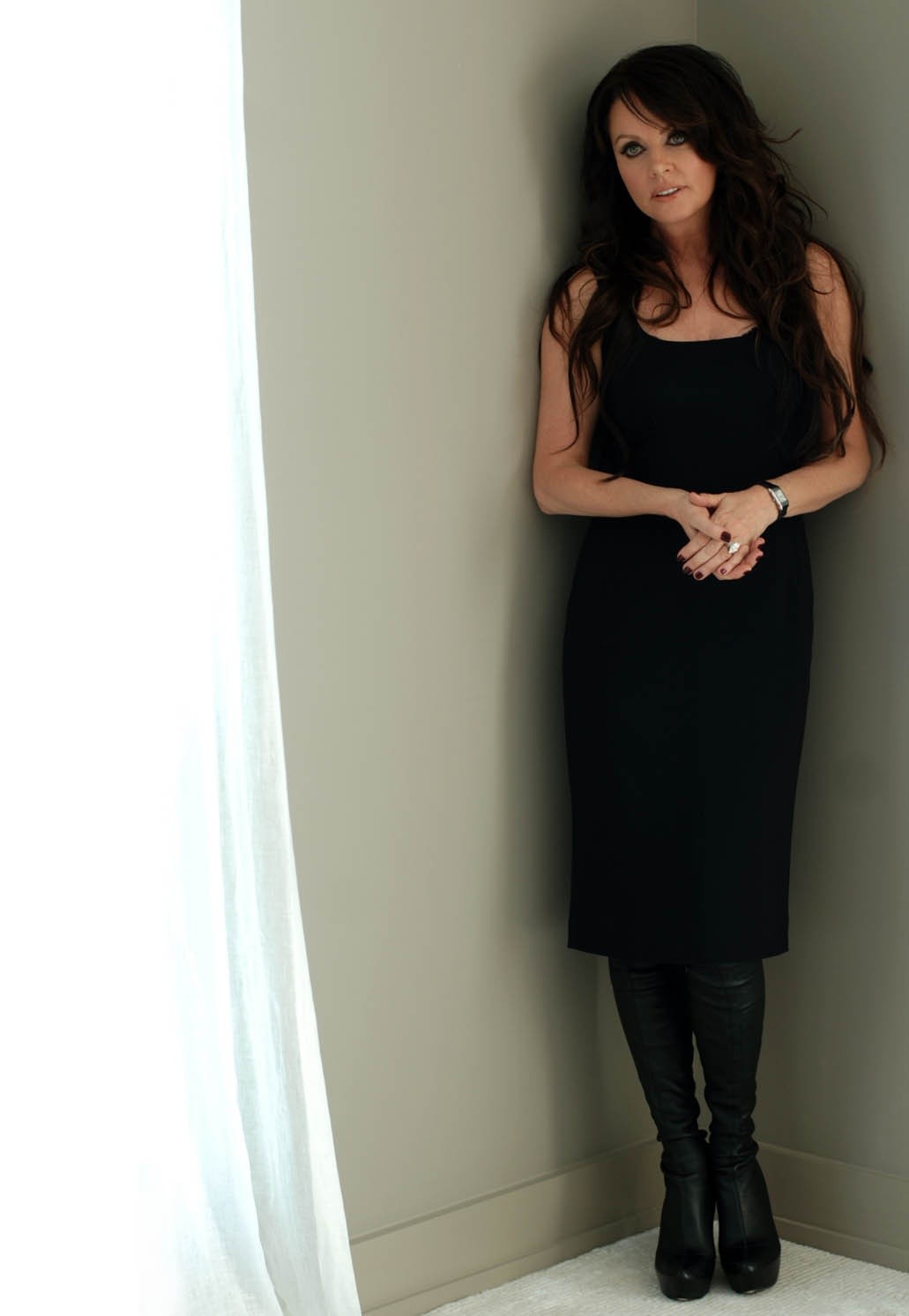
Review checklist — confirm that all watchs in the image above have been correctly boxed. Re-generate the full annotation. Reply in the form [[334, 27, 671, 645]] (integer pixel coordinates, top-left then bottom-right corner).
[[755, 480, 788, 522]]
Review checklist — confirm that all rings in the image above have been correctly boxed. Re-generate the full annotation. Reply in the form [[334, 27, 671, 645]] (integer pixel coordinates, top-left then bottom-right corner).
[[723, 541, 740, 555]]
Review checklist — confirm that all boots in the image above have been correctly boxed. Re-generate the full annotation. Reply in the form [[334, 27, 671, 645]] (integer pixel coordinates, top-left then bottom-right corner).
[[607, 952, 713, 1301], [691, 959, 780, 1296]]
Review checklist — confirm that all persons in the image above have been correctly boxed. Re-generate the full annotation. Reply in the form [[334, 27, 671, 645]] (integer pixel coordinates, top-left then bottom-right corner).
[[530, 41, 889, 1314]]
